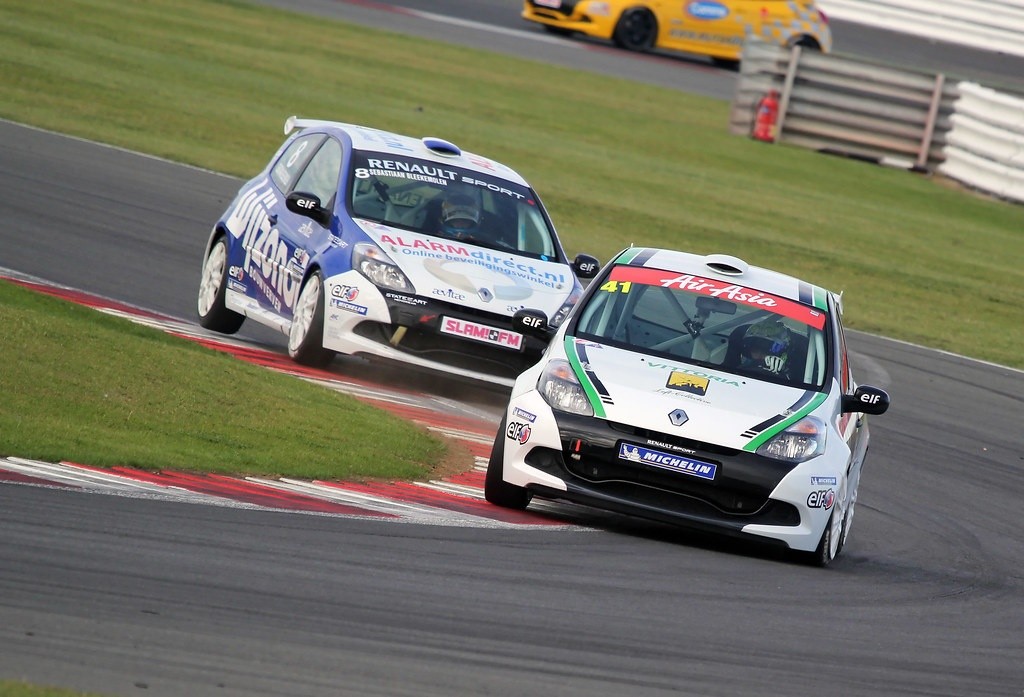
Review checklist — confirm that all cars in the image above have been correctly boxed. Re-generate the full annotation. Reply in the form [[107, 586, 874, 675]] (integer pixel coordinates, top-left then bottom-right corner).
[[477, 239, 895, 570], [193, 112, 601, 389], [521, 0, 833, 80]]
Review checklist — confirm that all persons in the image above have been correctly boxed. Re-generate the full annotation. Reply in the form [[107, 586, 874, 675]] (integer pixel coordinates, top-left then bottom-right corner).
[[435, 195, 484, 241], [735, 317, 797, 382]]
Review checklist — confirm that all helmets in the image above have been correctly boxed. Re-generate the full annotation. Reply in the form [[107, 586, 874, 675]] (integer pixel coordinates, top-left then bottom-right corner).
[[740, 323, 792, 372], [438, 192, 480, 238]]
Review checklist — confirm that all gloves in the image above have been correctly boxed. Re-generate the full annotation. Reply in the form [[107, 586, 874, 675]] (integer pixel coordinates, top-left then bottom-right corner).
[[762, 355, 785, 374]]
